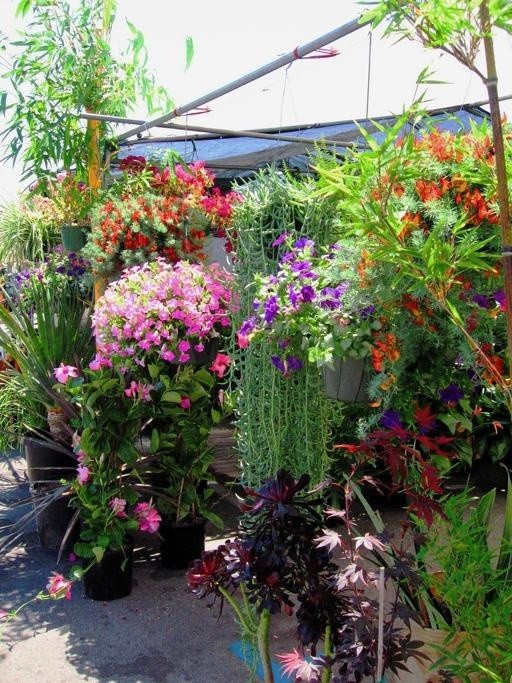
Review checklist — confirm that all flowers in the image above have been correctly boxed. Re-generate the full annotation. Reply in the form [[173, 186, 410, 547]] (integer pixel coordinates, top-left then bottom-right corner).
[[190, 469, 441, 683], [316, 113, 511, 500], [87, 153, 232, 264], [403, 495, 512, 683]]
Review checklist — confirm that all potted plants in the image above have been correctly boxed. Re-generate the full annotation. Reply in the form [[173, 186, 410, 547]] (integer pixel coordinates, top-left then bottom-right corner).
[[1, 238, 98, 551], [3, 357, 161, 621], [238, 229, 394, 402], [87, 263, 237, 570], [44, 176, 90, 252]]
